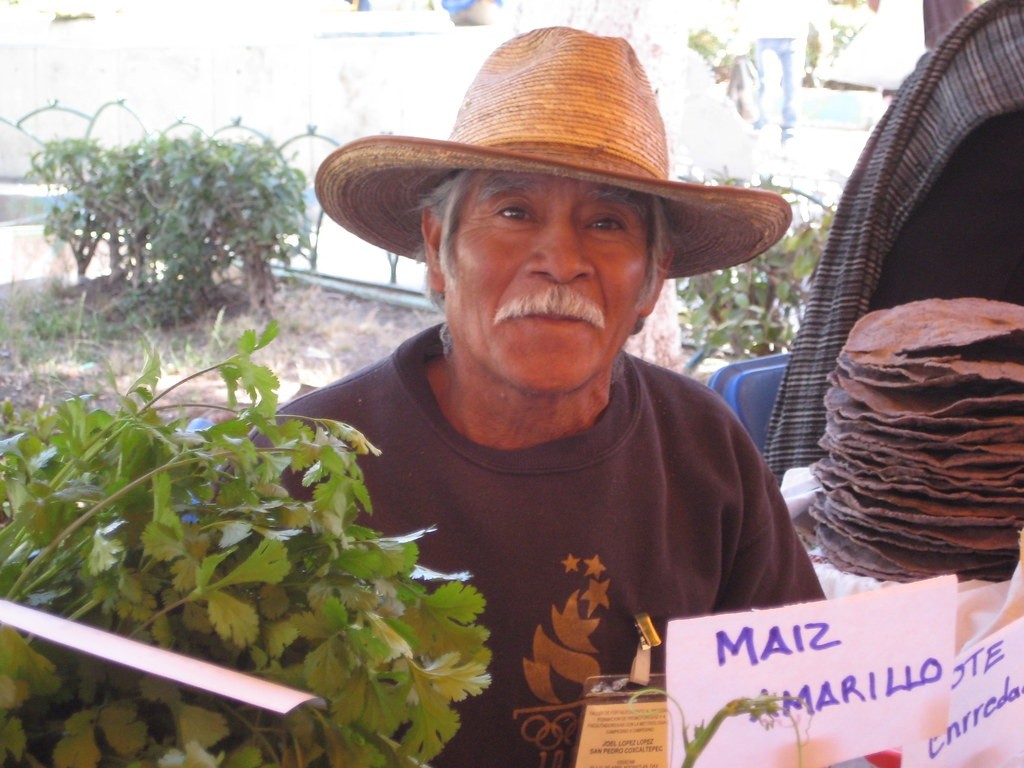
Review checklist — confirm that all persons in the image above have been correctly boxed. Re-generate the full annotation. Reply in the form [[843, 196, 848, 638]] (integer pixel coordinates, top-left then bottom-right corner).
[[753, 37, 800, 141], [214, 27, 826, 768], [761, 0, 1024, 486]]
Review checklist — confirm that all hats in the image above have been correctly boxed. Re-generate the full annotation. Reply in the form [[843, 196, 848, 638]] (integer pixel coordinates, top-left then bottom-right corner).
[[314, 27, 792, 277]]
